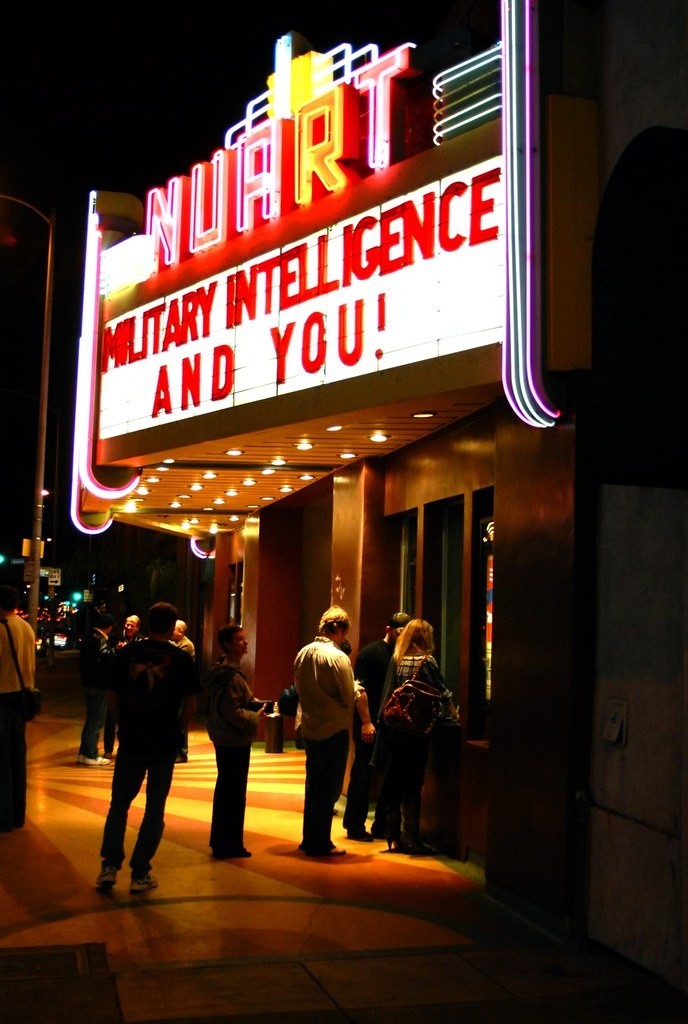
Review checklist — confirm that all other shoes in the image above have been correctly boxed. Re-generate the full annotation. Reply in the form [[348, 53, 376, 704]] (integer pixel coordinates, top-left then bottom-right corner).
[[83, 755, 111, 766], [106, 753, 113, 759], [346, 830, 377, 842], [174, 756, 188, 763], [77, 754, 85, 764], [304, 848, 346, 859], [95, 865, 117, 891], [129, 872, 159, 891]]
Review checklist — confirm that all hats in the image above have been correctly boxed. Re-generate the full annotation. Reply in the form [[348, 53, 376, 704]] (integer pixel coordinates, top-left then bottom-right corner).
[[98, 612, 118, 626]]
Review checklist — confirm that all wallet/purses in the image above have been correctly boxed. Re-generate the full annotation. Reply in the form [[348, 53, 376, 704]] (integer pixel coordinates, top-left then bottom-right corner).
[[248, 699, 275, 713]]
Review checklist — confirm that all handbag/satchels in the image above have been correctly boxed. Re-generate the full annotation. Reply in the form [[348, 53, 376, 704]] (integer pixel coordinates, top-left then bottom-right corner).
[[19, 686, 44, 721], [383, 654, 446, 736], [277, 684, 299, 716]]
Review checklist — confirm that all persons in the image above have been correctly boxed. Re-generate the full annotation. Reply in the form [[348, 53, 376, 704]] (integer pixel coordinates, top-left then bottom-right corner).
[[170, 620, 194, 763], [294, 605, 357, 856], [203, 626, 280, 858], [79, 614, 125, 766], [103, 615, 144, 758], [0, 589, 36, 831], [376, 619, 461, 855], [294, 640, 376, 814], [94, 603, 197, 892], [343, 612, 412, 842]]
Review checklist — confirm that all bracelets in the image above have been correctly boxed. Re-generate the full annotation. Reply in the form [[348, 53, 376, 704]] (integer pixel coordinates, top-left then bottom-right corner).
[[453, 715, 459, 721]]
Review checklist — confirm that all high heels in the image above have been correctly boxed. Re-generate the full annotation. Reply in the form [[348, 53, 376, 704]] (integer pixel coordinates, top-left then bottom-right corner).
[[383, 832, 404, 852]]
